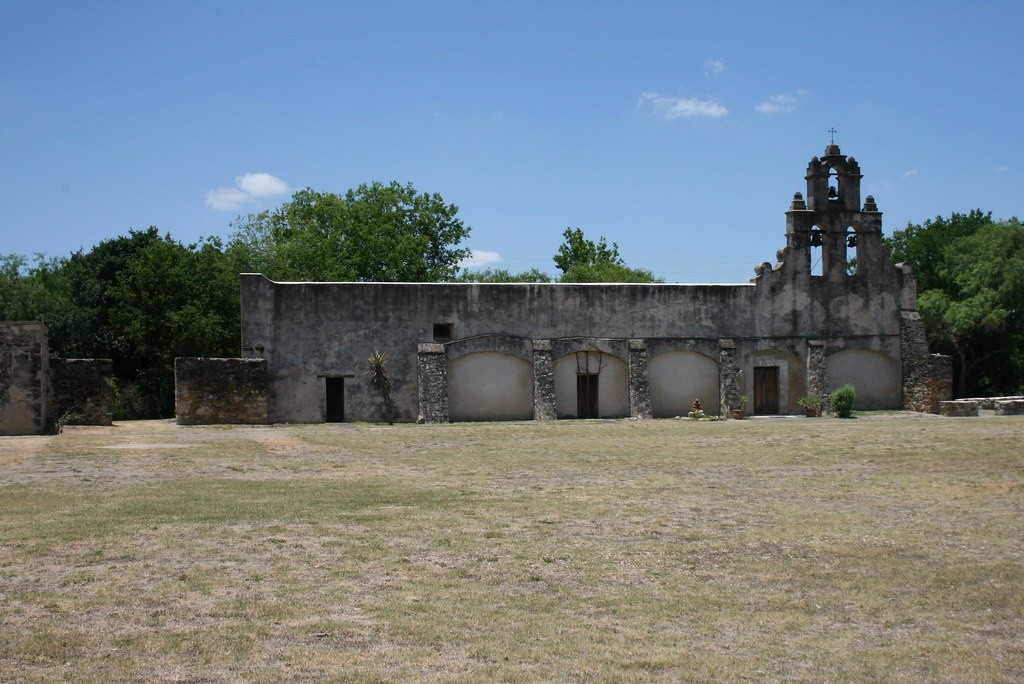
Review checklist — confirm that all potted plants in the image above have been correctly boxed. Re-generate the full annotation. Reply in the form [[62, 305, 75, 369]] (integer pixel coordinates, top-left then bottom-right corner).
[[795, 394, 825, 417], [732, 395, 751, 419]]
